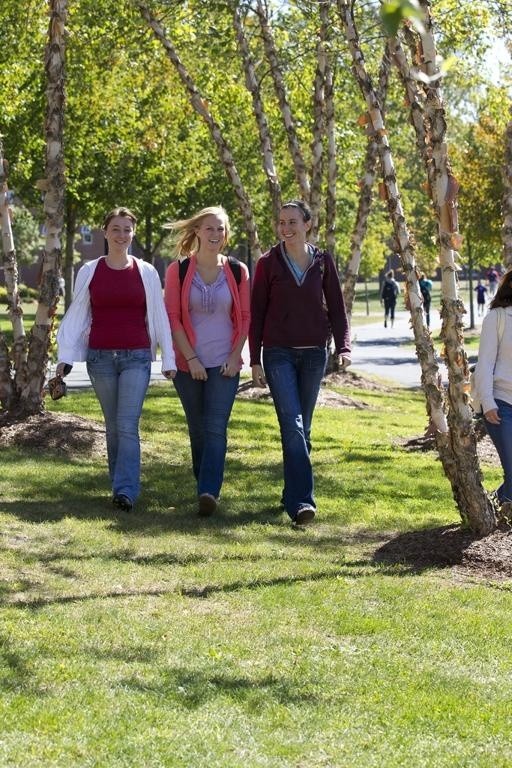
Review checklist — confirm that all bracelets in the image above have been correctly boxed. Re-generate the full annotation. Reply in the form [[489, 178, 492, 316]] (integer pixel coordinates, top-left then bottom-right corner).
[[187, 356, 198, 362]]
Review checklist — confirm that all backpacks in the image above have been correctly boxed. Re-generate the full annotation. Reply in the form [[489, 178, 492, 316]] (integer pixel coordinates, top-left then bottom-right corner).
[[419, 280, 432, 307], [382, 280, 396, 298]]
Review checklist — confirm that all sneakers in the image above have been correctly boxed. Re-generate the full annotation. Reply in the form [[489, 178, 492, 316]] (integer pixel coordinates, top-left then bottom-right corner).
[[196, 491, 217, 518], [384, 320, 388, 328], [294, 503, 316, 526], [111, 492, 135, 514]]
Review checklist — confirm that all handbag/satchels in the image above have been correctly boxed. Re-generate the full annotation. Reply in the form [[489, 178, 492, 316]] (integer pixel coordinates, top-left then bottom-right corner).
[[469, 363, 486, 420]]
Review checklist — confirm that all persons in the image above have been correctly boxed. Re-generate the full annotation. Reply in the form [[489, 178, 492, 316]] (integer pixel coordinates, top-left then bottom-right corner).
[[165, 207, 251, 516], [475, 270, 512, 506], [380, 270, 400, 328], [419, 266, 499, 326], [56, 207, 177, 512], [248, 201, 351, 529]]
